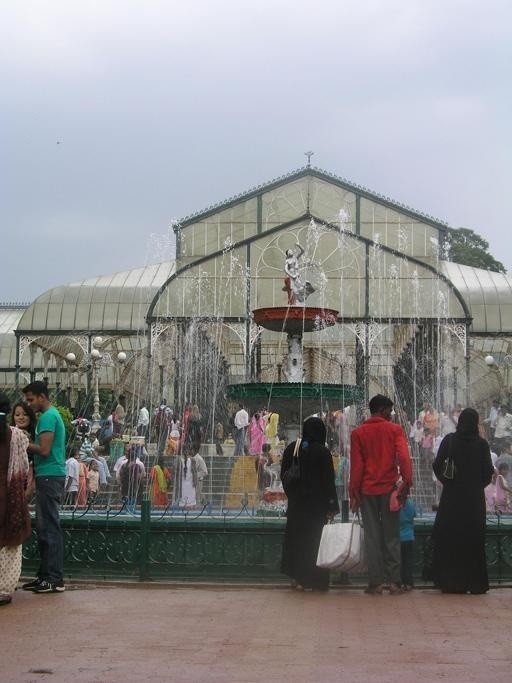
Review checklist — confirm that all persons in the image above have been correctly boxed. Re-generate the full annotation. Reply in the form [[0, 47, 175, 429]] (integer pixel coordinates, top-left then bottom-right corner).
[[397, 481, 417, 588], [0, 388, 35, 607], [286, 241, 304, 296], [282, 419, 339, 592], [1, 395, 512, 516], [21, 380, 68, 594], [433, 408, 489, 594], [350, 396, 413, 594]]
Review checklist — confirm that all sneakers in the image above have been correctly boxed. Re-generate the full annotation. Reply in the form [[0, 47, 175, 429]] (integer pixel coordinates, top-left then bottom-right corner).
[[34, 577, 66, 592], [23, 578, 42, 590]]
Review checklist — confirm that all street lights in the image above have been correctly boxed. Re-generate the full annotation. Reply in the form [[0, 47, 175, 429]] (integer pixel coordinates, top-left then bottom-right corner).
[[67, 336, 127, 432], [485, 354, 511, 397]]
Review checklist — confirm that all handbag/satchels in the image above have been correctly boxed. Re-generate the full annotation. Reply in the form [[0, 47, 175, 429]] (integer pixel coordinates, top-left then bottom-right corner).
[[315, 523, 367, 574], [434, 457, 456, 481]]
